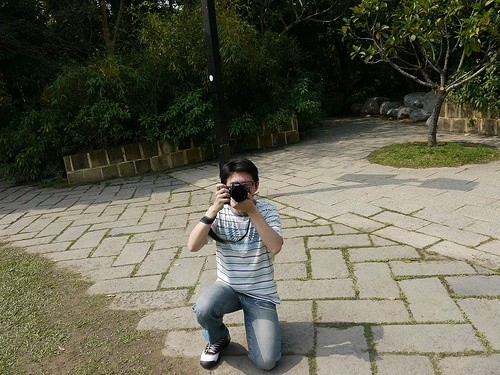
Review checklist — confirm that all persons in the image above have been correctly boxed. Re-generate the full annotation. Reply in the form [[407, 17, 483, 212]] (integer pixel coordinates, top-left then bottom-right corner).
[[186, 157, 285, 370]]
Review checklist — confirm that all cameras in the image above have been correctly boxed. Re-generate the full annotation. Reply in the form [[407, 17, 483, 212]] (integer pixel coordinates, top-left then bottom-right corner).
[[223, 182, 249, 204]]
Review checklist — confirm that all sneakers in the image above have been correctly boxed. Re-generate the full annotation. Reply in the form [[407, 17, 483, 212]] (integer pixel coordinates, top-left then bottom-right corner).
[[200, 333, 231, 370]]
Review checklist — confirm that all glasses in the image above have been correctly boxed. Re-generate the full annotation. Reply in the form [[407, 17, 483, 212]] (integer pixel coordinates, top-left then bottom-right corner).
[[240, 181, 256, 189]]
